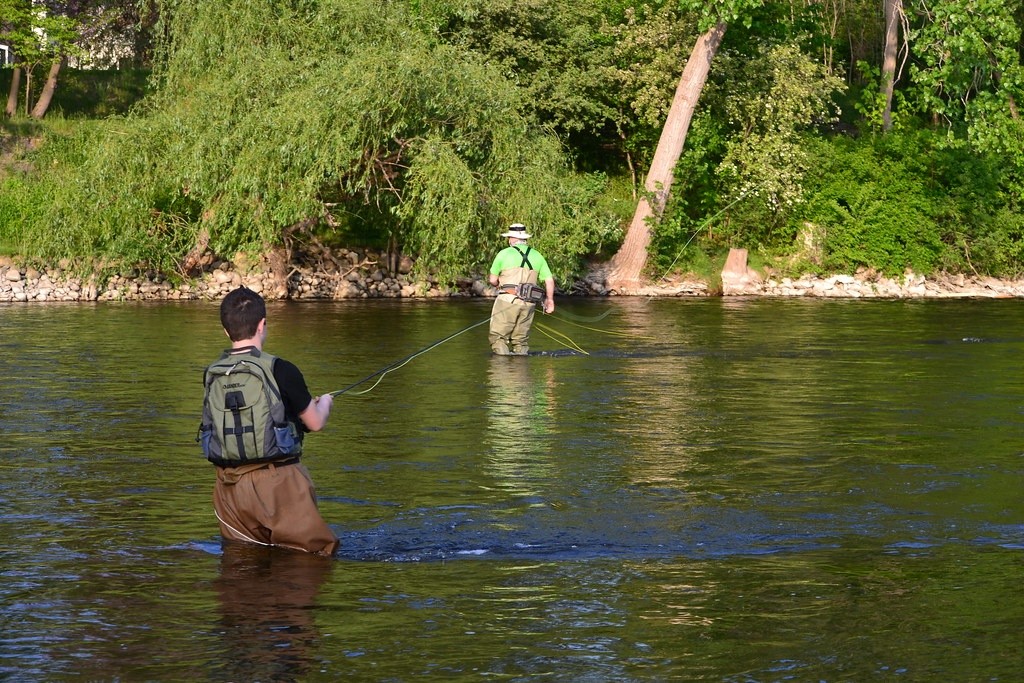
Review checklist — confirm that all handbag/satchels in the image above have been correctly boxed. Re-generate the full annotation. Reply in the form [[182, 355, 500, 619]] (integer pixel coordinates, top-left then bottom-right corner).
[[517, 284, 546, 303]]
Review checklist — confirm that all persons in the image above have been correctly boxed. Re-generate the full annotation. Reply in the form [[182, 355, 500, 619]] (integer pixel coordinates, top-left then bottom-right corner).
[[489, 223, 555, 357], [204, 286, 340, 558]]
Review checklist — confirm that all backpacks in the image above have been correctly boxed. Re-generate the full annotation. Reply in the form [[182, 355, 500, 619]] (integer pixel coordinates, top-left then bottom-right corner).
[[196, 345, 304, 468]]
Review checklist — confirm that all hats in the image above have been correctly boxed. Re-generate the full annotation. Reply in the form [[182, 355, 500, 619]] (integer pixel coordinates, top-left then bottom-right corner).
[[500, 224, 532, 239]]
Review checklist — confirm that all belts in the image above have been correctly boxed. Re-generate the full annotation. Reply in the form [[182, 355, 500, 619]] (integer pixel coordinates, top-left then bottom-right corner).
[[258, 456, 300, 469]]
